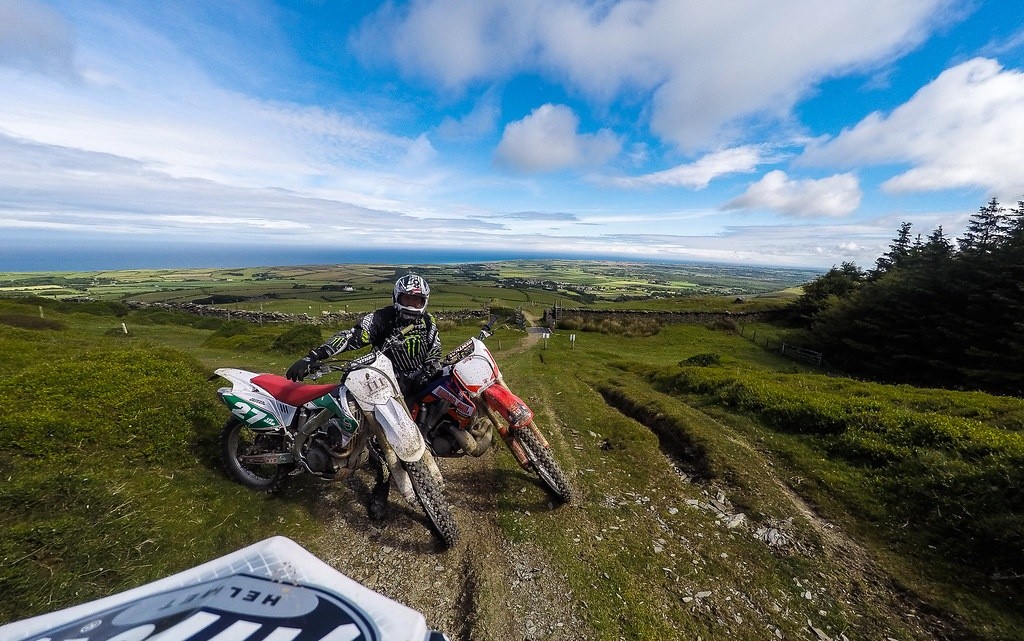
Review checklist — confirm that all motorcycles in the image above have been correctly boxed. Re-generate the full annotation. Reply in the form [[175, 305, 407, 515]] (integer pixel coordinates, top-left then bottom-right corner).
[[407, 314, 571, 504], [206, 322, 461, 552]]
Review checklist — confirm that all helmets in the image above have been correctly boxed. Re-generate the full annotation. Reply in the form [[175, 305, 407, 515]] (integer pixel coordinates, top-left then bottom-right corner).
[[392, 274, 430, 322]]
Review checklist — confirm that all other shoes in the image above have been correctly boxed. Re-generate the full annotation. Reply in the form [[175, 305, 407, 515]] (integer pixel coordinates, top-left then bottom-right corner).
[[368, 477, 390, 522]]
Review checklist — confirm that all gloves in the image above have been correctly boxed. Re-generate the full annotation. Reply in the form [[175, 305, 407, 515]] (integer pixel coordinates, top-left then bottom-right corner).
[[404, 364, 426, 389], [286, 347, 329, 382]]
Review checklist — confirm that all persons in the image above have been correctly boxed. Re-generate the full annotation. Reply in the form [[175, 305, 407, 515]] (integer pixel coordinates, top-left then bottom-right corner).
[[285, 276, 442, 519]]
